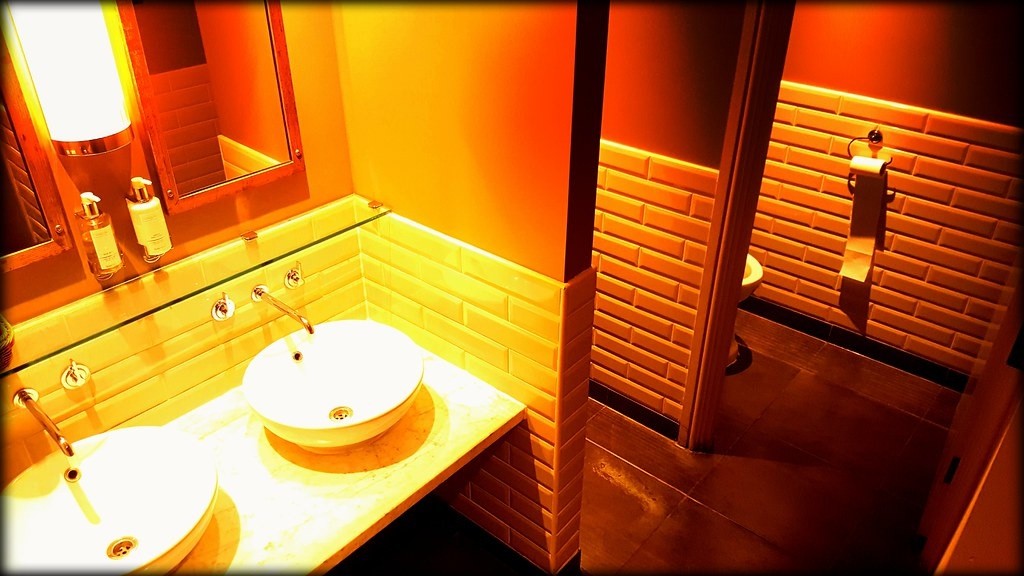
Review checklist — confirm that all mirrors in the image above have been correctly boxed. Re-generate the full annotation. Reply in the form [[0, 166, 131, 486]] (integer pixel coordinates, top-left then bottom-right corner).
[[0, 26, 74, 275], [114, 0, 306, 217]]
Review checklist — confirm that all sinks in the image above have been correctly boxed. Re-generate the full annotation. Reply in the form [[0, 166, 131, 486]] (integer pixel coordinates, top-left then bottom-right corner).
[[242, 319, 425, 456], [0, 427, 218, 576]]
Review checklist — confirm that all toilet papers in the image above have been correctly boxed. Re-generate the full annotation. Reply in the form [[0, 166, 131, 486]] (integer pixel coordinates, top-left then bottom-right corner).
[[837, 155, 888, 284]]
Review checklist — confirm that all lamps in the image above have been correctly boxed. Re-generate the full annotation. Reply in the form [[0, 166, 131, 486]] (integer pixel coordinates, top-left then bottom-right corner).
[[7, 0, 135, 157]]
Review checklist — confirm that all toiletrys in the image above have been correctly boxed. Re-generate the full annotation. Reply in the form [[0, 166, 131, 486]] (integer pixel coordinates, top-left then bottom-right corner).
[[80, 191, 125, 274], [125, 177, 172, 255]]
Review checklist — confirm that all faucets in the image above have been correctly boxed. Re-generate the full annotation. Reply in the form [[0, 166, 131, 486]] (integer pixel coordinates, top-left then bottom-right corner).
[[12, 388, 75, 457], [251, 284, 316, 335]]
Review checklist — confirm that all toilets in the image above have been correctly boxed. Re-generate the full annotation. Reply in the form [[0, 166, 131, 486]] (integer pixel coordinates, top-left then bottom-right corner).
[[726, 252, 764, 366]]
